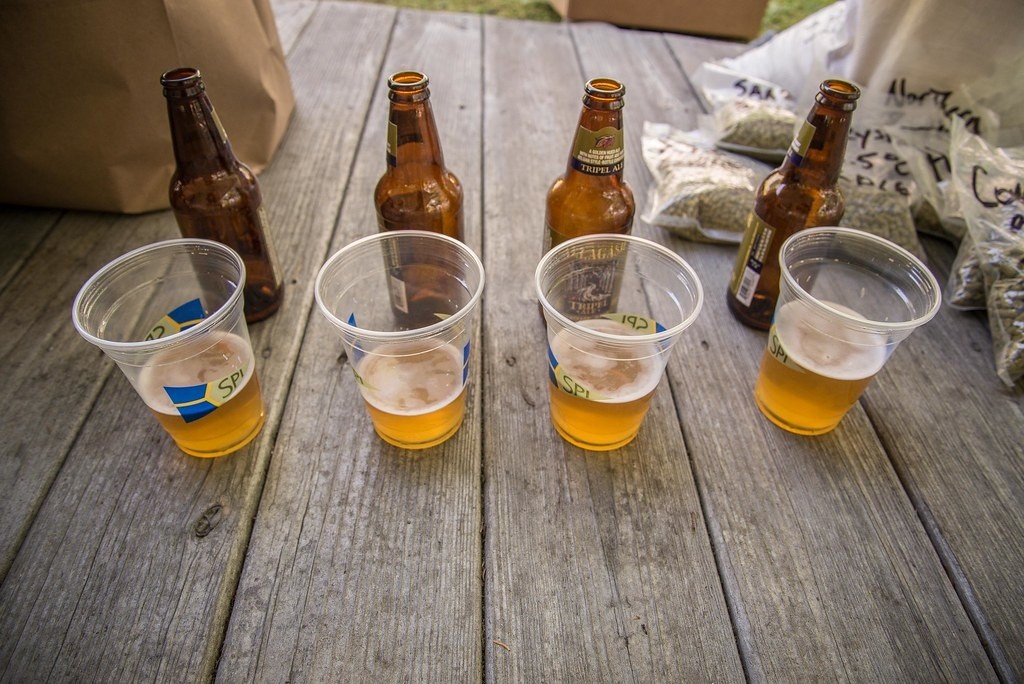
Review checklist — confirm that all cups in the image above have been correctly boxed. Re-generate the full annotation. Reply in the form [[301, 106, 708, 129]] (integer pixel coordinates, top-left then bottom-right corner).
[[71, 240, 265, 458], [313, 230, 485, 448], [754, 227, 941, 435], [536, 233, 703, 451]]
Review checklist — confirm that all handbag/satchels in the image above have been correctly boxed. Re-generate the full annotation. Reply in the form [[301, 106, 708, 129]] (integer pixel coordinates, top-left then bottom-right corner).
[[0, 1, 295, 213]]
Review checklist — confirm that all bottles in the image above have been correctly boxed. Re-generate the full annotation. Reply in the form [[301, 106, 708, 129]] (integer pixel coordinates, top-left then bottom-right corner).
[[726, 81, 860, 332], [160, 67, 282, 326], [537, 79, 635, 327], [374, 73, 465, 327]]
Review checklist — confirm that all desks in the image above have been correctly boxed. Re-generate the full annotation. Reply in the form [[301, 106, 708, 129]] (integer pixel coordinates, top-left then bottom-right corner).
[[0, 0, 1024, 684]]
